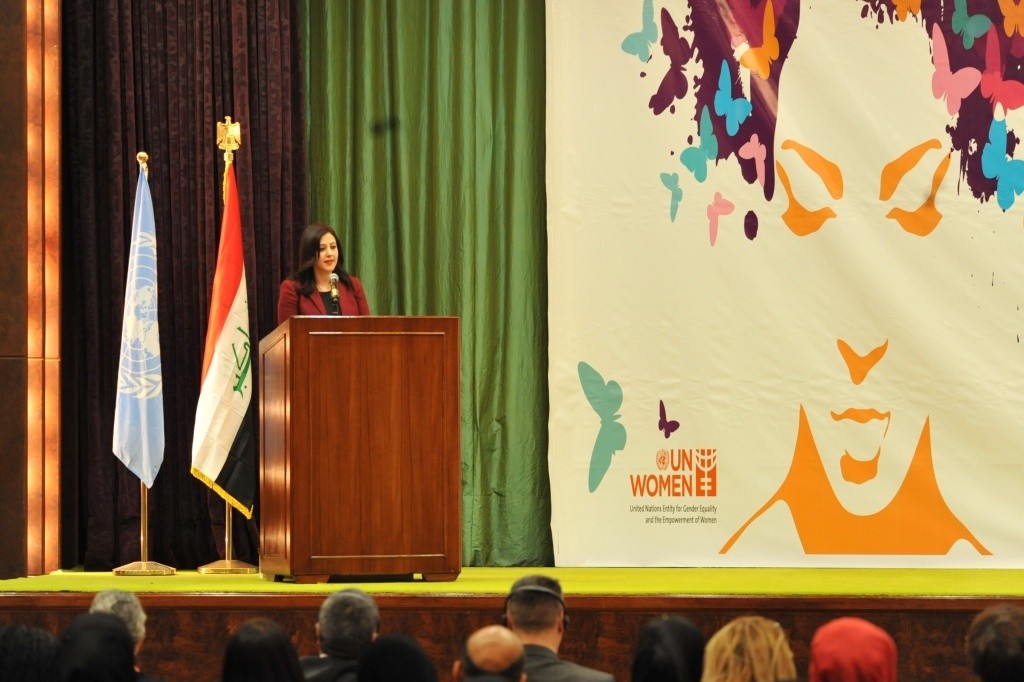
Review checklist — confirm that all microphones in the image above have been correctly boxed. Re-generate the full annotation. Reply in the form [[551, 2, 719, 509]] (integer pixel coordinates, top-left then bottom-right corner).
[[328, 273, 339, 303]]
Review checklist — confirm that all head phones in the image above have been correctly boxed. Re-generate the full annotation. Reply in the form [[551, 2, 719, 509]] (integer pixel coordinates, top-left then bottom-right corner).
[[500, 584, 570, 630]]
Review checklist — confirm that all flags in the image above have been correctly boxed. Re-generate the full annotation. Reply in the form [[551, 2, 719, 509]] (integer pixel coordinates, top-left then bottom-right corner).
[[188, 163, 260, 522], [108, 166, 167, 488]]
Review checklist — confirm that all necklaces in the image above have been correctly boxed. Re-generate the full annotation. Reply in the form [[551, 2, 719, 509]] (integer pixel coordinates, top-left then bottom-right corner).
[[316, 282, 331, 301]]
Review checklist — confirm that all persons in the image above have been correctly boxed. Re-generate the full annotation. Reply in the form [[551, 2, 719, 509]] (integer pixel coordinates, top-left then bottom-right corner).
[[699, 616, 797, 682], [502, 577, 617, 682], [1, 591, 147, 682], [218, 617, 304, 682], [629, 615, 708, 682], [357, 633, 438, 682], [808, 615, 898, 682], [297, 588, 379, 682], [276, 224, 372, 327], [961, 603, 1024, 682], [451, 623, 529, 682]]
